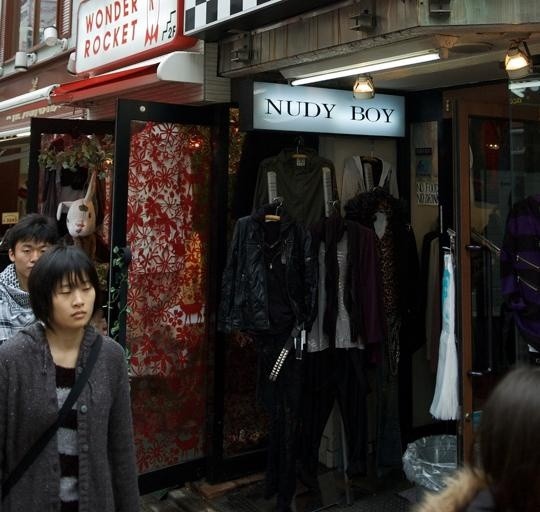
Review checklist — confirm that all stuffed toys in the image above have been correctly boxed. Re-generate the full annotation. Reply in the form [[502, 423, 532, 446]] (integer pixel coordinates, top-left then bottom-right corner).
[[56, 171, 98, 259]]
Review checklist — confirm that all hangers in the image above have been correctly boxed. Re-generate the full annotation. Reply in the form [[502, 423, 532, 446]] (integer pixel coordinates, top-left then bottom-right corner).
[[363, 151, 378, 162], [265, 198, 282, 221], [292, 137, 307, 158]]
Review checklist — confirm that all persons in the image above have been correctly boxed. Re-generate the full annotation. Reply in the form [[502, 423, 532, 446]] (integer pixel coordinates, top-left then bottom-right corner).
[[1, 244, 141, 511], [0, 212, 60, 344], [419, 365, 540, 511]]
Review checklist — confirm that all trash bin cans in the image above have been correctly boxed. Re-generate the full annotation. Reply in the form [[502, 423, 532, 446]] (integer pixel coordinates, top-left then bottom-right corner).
[[402, 434, 457, 503]]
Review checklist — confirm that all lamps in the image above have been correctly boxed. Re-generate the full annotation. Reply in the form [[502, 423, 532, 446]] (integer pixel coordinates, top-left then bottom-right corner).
[[67, 51, 78, 76], [43, 26, 68, 51], [508, 80, 540, 98], [288, 48, 442, 88], [353, 73, 376, 100], [505, 38, 533, 79], [15, 52, 37, 72]]
